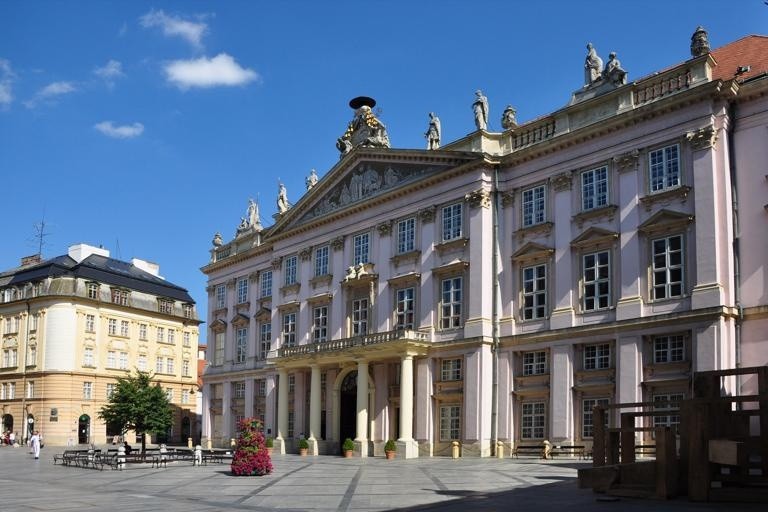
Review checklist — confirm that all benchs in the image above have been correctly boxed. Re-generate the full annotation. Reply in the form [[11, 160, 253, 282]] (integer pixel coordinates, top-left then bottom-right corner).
[[511, 445, 656, 461], [53, 449, 236, 472]]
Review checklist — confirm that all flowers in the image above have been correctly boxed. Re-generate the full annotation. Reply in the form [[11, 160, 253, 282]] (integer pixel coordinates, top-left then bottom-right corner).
[[230, 418, 273, 476]]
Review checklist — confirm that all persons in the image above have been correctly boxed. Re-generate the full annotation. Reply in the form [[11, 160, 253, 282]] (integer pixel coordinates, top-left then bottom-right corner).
[[244, 198, 263, 233], [29, 430, 43, 460], [424, 112, 443, 152], [294, 198, 333, 226], [604, 51, 625, 82], [276, 183, 290, 215], [234, 216, 249, 240], [471, 88, 488, 130], [501, 105, 517, 129], [209, 231, 223, 264], [583, 42, 604, 83], [331, 162, 417, 208], [304, 168, 318, 191], [0, 429, 16, 446], [335, 104, 392, 158], [123, 440, 132, 454]]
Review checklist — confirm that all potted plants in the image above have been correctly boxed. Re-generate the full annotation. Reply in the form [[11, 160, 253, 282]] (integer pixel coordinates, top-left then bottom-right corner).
[[298, 439, 309, 457], [342, 438, 354, 458], [265, 439, 273, 456], [384, 440, 396, 460]]
